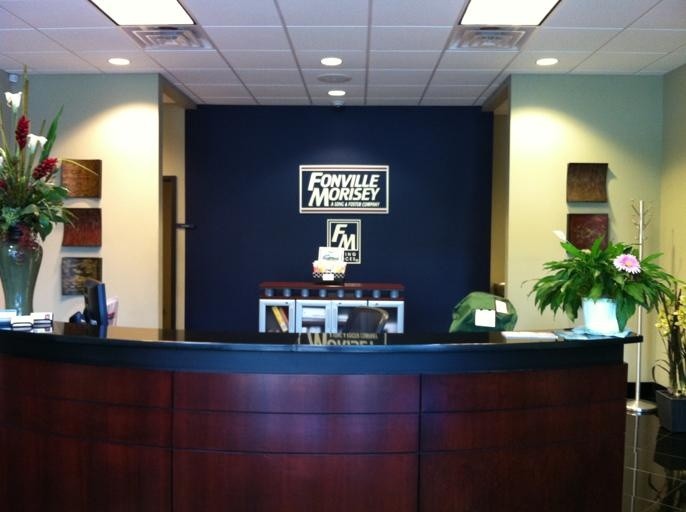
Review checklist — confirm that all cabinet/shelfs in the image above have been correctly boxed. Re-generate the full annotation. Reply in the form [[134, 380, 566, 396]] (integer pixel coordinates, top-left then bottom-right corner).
[[258, 282, 406, 333]]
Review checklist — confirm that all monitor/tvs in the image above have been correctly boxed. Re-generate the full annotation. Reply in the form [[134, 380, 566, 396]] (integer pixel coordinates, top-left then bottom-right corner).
[[71, 277, 108, 325]]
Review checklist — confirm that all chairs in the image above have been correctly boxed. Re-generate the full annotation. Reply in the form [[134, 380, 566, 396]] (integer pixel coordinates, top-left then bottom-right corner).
[[343, 303, 390, 333]]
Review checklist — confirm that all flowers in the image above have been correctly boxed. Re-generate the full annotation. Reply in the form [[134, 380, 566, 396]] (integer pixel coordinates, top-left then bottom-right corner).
[[651, 280, 686, 398], [520, 230, 680, 332], [0, 64, 99, 242]]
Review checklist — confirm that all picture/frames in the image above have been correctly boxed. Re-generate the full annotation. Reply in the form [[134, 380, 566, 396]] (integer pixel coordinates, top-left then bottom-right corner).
[[565, 162, 611, 250], [61, 158, 103, 295]]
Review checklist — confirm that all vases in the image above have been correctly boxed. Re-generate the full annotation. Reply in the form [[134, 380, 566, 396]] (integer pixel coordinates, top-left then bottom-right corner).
[[580, 297, 616, 336], [0, 243, 44, 315], [655, 391, 686, 433]]
